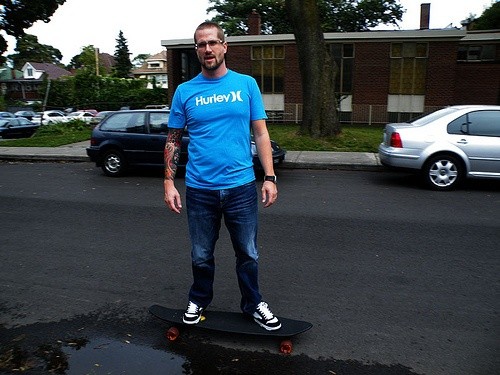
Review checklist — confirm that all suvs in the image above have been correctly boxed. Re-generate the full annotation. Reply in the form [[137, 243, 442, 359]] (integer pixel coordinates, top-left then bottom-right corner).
[[85, 110, 286, 177], [145, 105, 168, 110]]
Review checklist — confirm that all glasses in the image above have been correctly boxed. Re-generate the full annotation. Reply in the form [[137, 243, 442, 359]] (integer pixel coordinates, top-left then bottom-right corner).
[[195, 39, 223, 47]]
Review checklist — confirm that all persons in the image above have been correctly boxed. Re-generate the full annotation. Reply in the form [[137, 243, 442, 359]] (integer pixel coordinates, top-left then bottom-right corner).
[[163, 21, 280, 329]]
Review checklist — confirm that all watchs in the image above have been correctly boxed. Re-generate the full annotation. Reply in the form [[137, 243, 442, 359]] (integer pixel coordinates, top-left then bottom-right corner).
[[264, 175, 277, 183]]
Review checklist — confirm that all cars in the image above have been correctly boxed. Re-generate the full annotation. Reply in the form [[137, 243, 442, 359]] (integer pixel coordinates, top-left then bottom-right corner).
[[31, 111, 71, 125], [14, 110, 41, 120], [0, 112, 16, 118], [0, 117, 41, 139], [65, 111, 95, 124], [377, 105, 500, 191], [89, 110, 114, 126]]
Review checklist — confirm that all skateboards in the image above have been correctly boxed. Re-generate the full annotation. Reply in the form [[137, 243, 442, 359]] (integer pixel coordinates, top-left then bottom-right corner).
[[150, 304, 313, 354]]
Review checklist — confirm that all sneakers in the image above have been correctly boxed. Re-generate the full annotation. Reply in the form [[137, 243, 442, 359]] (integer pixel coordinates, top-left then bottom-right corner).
[[240, 298, 281, 330], [183, 297, 207, 324]]
